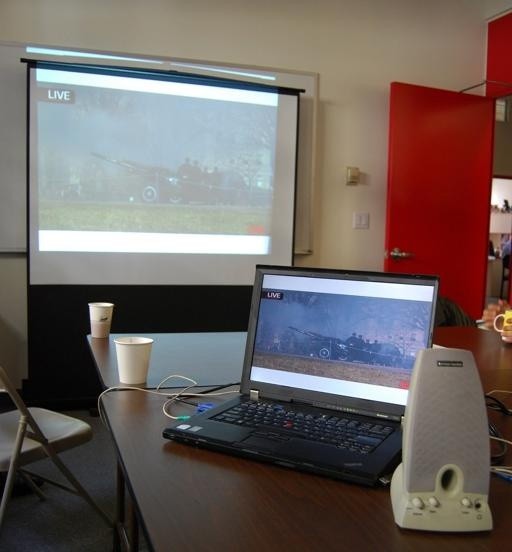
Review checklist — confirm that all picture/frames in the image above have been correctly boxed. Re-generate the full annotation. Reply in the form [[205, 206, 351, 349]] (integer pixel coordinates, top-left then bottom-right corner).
[[86, 321, 511, 390], [98, 368, 512, 551]]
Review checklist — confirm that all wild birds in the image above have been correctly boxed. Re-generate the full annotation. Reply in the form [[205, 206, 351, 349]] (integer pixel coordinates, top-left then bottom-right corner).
[[163, 264, 440, 488]]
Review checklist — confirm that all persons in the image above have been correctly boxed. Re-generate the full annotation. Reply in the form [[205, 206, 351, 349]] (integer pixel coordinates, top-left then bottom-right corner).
[[179, 158, 226, 189], [501, 238, 512, 271], [481, 290, 511, 346], [345, 331, 380, 353]]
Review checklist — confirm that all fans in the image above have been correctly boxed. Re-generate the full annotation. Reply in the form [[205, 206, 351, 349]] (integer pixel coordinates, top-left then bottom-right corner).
[[1, 42, 318, 255]]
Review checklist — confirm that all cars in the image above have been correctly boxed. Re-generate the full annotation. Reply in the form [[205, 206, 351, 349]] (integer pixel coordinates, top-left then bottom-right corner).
[[113, 335, 154, 385], [493, 309, 512, 343], [87, 301, 114, 338]]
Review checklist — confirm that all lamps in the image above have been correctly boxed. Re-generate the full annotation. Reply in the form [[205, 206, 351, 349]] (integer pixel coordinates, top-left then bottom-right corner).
[[0, 366, 116, 532], [499, 253, 512, 301]]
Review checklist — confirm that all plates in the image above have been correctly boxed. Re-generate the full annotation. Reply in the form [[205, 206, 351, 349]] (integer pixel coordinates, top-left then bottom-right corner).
[[488, 175, 512, 259]]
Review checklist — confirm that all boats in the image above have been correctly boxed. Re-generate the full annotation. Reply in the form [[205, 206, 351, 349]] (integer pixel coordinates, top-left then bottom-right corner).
[[390, 348, 495, 533]]
